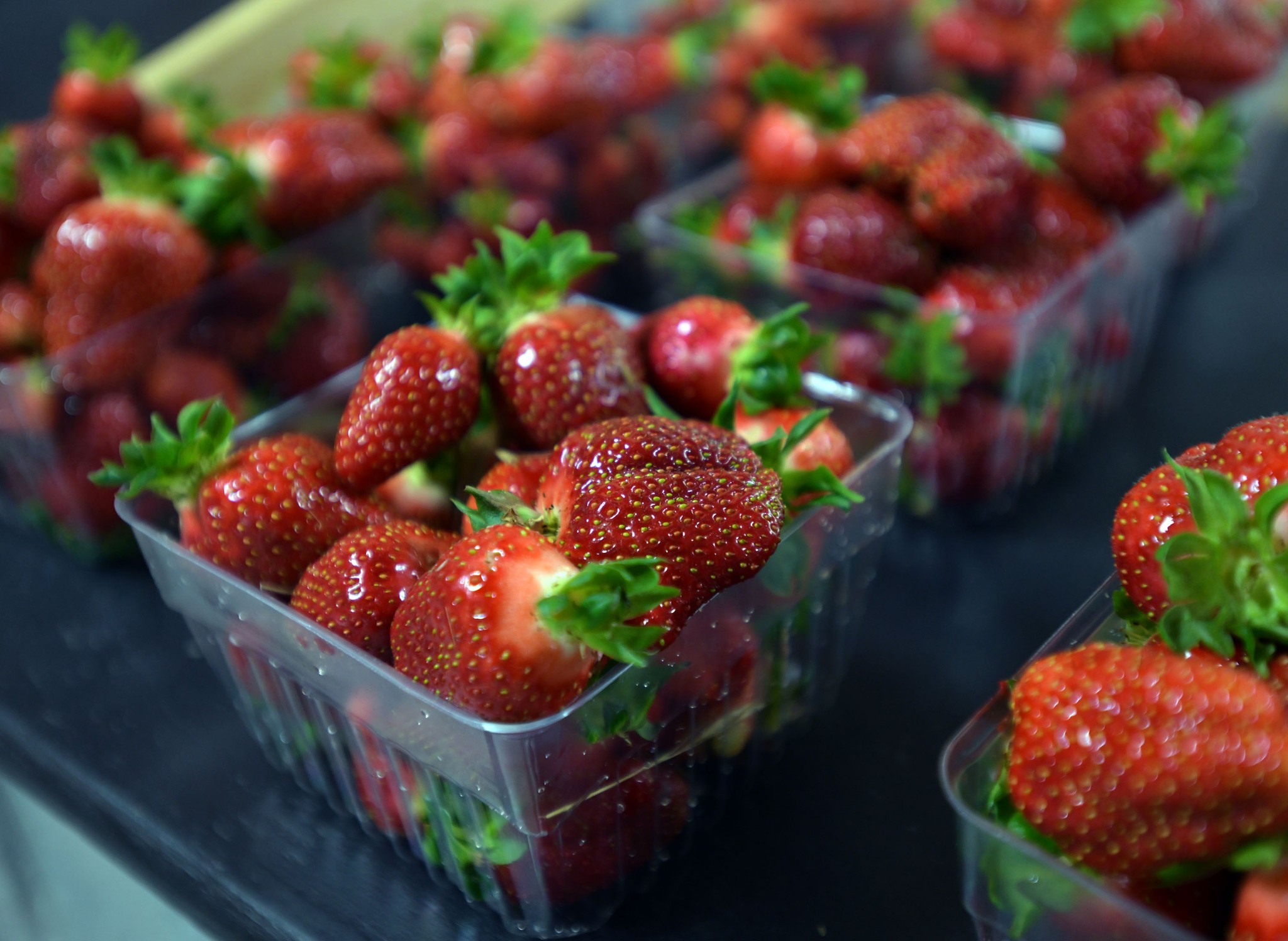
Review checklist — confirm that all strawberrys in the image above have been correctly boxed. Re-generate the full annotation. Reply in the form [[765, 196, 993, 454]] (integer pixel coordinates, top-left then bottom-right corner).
[[972, 413, 1288, 941], [97, 226, 875, 932], [1, 1, 899, 552], [392, 0, 1288, 514]]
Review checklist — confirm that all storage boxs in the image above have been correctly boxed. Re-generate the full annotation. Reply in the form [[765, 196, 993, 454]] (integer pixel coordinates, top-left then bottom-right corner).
[[107, 276, 913, 941], [640, 95, 1187, 516], [940, 576, 1225, 941]]
